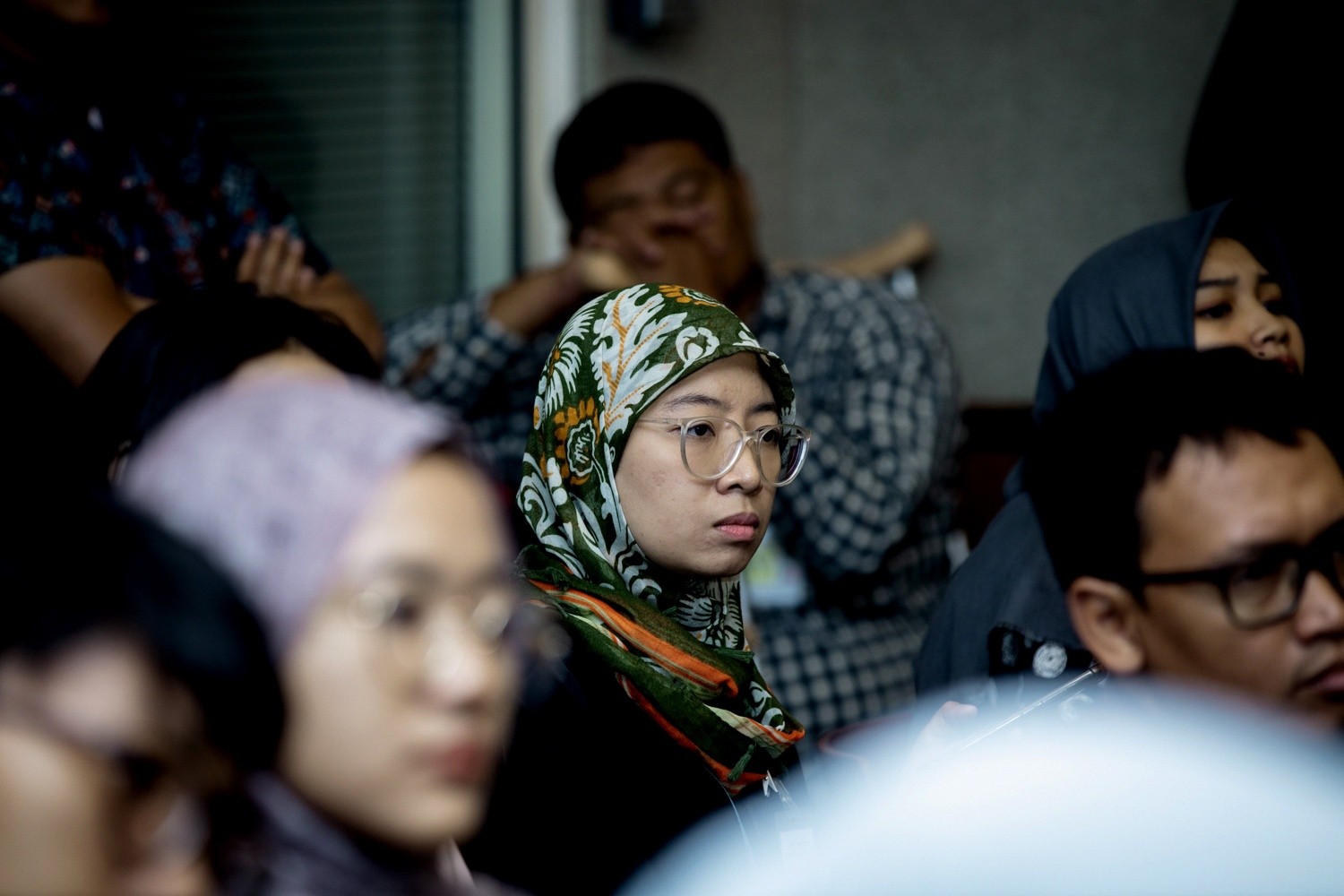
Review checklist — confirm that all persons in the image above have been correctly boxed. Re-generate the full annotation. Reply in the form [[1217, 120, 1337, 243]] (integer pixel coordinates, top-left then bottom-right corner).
[[0, 1, 398, 497], [0, 463, 293, 896], [454, 280, 812, 896], [1031, 343, 1344, 744], [1181, 0, 1344, 409], [384, 74, 978, 761], [116, 375, 532, 896], [766, 223, 935, 288], [911, 218, 1306, 699]]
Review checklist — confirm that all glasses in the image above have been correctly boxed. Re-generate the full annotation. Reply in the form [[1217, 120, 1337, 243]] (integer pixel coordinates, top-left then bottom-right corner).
[[639, 413, 813, 489], [347, 585, 540, 679], [0, 678, 282, 895], [1116, 527, 1344, 632]]
